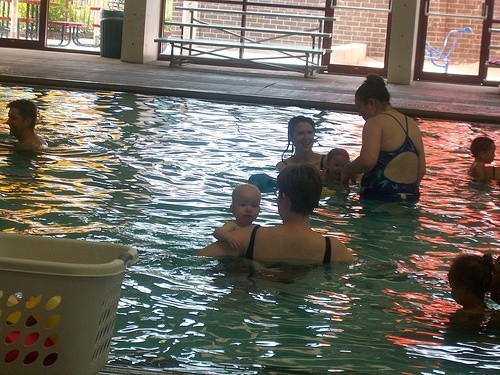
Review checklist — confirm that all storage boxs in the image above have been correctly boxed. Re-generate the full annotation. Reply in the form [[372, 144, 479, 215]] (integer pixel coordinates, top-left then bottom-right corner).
[[0, 232, 139, 375]]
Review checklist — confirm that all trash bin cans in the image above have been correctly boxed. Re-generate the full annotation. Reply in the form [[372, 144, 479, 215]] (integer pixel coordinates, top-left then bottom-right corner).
[[99, 8, 124, 58]]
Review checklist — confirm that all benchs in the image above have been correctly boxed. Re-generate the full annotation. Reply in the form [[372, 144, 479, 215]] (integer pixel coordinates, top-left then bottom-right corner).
[[154, 6, 337, 78], [0, 0, 103, 48]]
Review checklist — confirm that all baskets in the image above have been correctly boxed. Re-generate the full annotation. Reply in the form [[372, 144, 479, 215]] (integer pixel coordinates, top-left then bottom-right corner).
[[0, 233, 139, 375]]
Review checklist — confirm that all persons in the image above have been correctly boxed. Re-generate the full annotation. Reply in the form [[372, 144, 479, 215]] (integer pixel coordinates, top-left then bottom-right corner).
[[213, 184, 261, 252], [197, 164, 355, 262], [447, 251, 500, 310], [6, 99, 45, 145], [318, 147, 350, 171], [340, 78, 426, 193], [275, 116, 326, 167], [468, 137, 500, 179]]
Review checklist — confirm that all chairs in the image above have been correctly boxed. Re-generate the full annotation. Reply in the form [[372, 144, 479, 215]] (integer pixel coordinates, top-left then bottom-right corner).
[[424, 27, 472, 74]]
